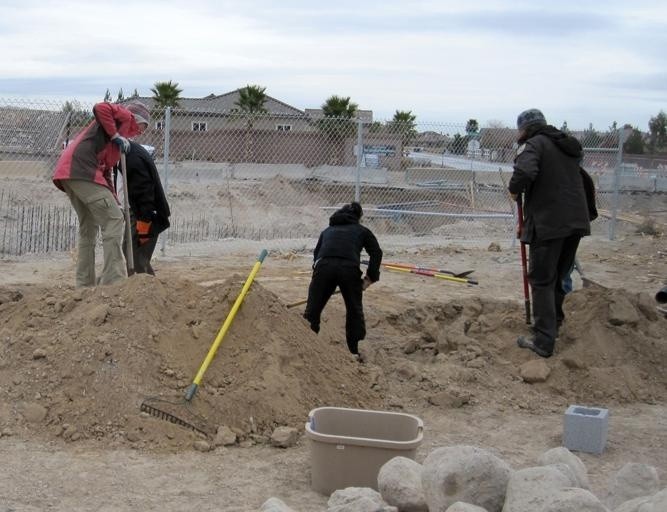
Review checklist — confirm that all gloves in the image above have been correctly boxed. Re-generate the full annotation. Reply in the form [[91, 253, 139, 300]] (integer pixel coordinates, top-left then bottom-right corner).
[[111, 132, 131, 155], [136, 220, 152, 244]]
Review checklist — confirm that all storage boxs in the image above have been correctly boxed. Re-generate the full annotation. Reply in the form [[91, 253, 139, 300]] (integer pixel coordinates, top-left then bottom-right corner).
[[305, 406, 423, 495]]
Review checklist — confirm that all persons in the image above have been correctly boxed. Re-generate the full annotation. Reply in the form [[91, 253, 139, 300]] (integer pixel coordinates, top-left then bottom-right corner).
[[111, 139, 171, 274], [302, 202, 382, 354], [52, 98, 150, 288], [507, 107, 591, 358], [554, 166, 599, 335]]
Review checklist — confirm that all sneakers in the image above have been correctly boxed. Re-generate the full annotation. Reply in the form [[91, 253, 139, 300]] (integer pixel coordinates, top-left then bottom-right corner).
[[518, 336, 552, 357]]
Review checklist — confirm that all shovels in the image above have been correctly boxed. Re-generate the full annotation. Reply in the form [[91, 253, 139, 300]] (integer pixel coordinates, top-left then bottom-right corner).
[[360, 259, 477, 278]]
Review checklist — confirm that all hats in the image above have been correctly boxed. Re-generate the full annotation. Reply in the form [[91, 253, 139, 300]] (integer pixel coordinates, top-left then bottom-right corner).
[[343, 203, 363, 220], [125, 100, 150, 129], [518, 109, 546, 131]]
